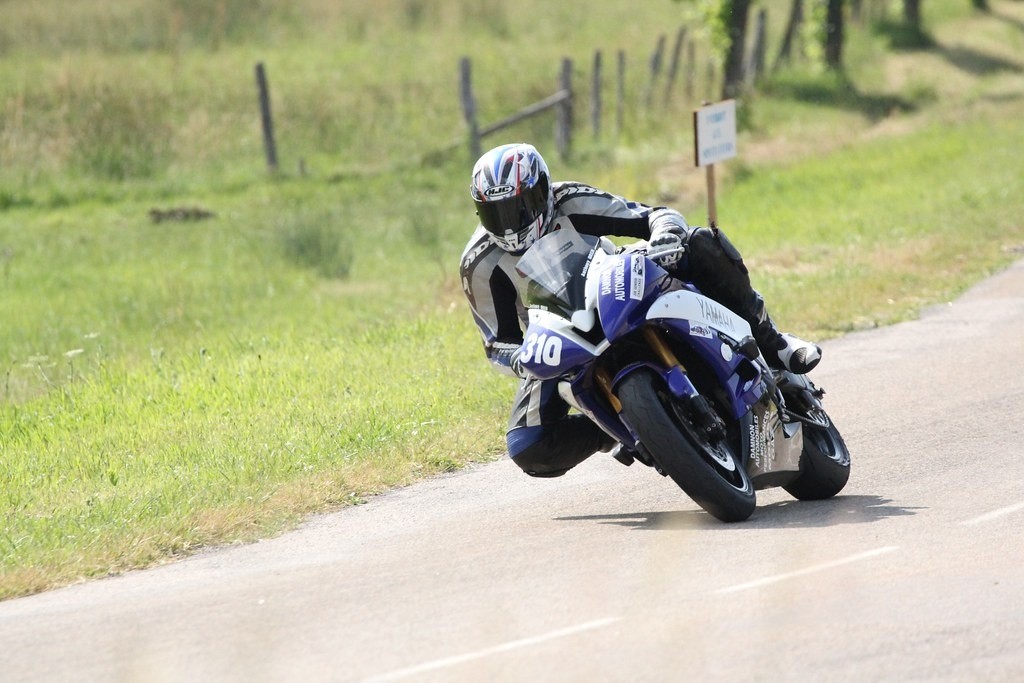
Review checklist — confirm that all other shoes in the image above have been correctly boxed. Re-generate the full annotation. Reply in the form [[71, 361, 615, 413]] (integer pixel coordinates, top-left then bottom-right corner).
[[777, 332, 823, 375]]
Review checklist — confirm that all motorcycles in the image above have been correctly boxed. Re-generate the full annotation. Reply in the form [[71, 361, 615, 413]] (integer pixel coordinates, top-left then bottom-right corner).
[[519, 227, 851, 523]]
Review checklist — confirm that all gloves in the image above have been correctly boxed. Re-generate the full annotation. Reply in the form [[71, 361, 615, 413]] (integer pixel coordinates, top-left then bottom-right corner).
[[646, 233, 686, 272], [510, 346, 537, 381]]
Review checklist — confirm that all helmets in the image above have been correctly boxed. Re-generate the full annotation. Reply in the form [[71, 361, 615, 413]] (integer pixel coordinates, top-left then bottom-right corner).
[[471, 142, 555, 257]]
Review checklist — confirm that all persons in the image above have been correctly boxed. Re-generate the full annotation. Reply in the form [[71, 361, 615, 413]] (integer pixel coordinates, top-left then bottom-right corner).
[[458, 143, 822, 479]]
